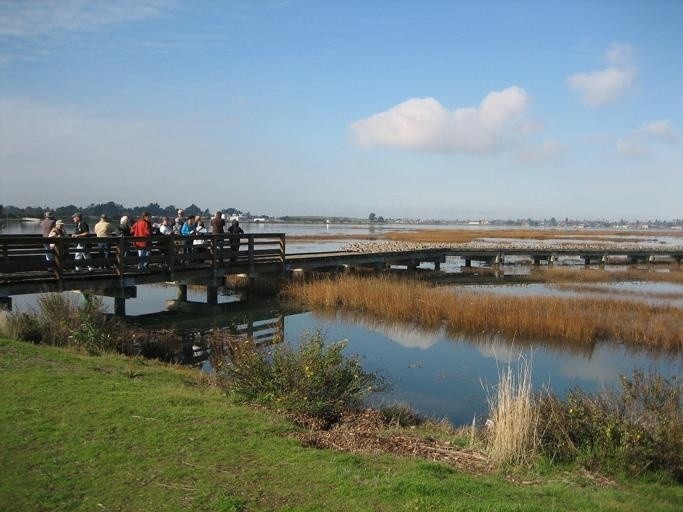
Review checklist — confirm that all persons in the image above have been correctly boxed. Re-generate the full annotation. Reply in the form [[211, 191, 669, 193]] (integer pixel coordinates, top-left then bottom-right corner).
[[69, 211, 95, 272], [37, 211, 56, 271], [45, 218, 68, 275], [91, 206, 226, 272], [227, 219, 244, 261]]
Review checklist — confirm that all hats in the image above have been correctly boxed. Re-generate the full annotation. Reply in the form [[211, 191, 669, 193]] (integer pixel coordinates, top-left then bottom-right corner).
[[55, 219, 65, 225], [45, 212, 54, 218], [71, 211, 81, 218], [101, 214, 107, 218]]
